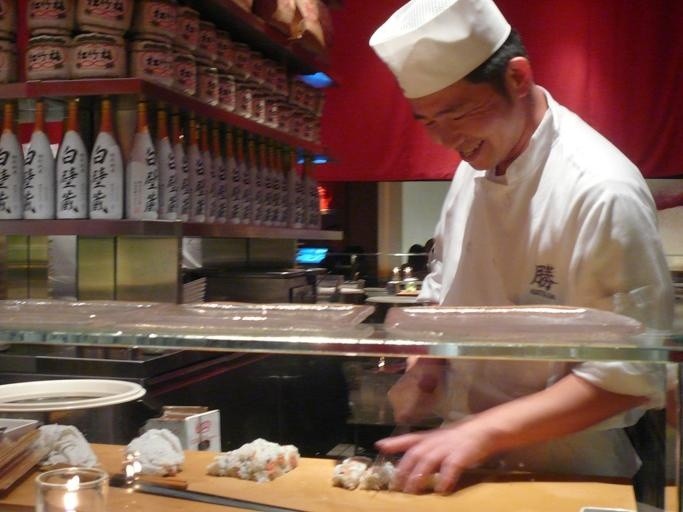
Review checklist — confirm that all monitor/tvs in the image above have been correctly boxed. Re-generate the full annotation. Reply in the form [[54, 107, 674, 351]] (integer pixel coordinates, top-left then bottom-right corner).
[[295, 245, 330, 264]]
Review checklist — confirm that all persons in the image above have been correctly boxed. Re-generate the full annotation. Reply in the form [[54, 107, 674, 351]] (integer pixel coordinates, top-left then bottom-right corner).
[[372, 26, 676, 510]]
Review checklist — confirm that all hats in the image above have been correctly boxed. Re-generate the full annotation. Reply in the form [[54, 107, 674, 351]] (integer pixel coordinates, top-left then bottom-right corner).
[[368, 0, 511, 99]]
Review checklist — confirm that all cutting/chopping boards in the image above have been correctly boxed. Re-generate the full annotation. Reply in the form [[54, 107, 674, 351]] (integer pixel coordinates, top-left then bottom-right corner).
[[0, 442, 640, 512]]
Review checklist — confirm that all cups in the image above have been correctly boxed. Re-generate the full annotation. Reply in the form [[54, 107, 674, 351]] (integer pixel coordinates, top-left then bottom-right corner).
[[30, 467, 111, 512]]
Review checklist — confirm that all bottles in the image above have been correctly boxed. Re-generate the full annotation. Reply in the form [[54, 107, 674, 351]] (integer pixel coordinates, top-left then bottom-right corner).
[[0, 98, 322, 233]]
